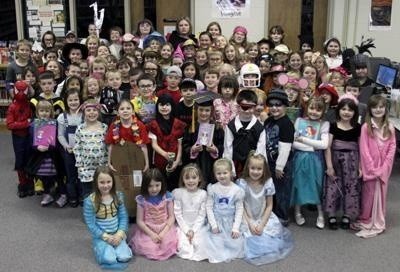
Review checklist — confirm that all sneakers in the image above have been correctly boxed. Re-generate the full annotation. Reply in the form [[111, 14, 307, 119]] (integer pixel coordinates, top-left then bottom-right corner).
[[295, 214, 305, 225], [316, 217, 325, 228], [18, 179, 44, 197], [80, 197, 85, 206], [340, 216, 351, 229], [71, 200, 79, 207], [41, 194, 54, 204], [277, 217, 289, 225], [57, 195, 67, 208], [328, 217, 337, 229]]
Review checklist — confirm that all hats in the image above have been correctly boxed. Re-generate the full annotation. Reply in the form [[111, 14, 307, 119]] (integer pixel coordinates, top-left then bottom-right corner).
[[166, 65, 182, 77], [190, 90, 222, 133], [267, 84, 289, 108], [63, 43, 88, 61], [179, 78, 196, 88], [66, 31, 76, 37], [234, 26, 247, 34], [257, 38, 292, 66], [119, 32, 196, 58], [262, 65, 290, 78], [319, 83, 338, 106], [32, 40, 45, 52]]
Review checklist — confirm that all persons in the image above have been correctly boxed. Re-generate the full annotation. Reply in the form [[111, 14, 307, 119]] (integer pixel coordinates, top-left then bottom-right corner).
[[6, 17, 397, 271]]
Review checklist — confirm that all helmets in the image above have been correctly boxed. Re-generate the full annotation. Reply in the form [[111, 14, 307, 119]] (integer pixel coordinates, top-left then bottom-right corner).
[[240, 64, 261, 87]]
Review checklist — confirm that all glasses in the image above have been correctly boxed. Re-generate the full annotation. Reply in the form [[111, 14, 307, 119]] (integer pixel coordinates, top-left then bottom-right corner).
[[267, 102, 285, 106]]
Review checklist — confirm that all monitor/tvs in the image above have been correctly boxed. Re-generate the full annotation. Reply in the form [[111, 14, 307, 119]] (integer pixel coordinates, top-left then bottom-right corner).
[[376, 64, 397, 88]]
